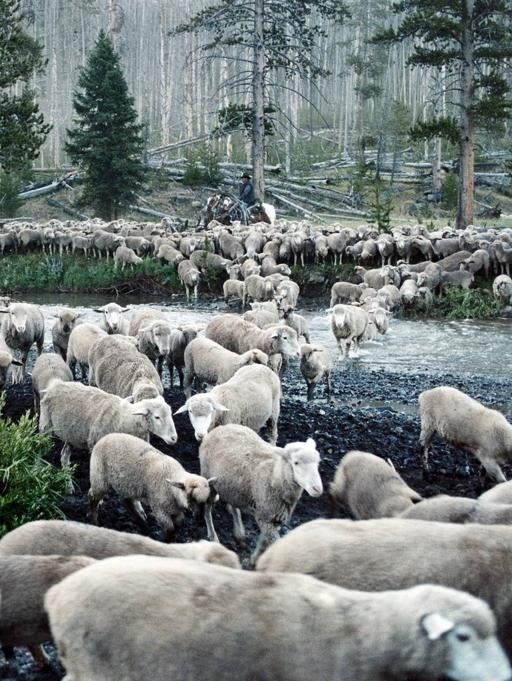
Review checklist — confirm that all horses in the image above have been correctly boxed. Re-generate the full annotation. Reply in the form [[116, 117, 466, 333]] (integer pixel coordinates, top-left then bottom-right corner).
[[198, 194, 278, 231]]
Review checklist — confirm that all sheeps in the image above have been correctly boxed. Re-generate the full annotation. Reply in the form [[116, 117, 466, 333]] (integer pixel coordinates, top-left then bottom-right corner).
[[0, 218, 512, 681]]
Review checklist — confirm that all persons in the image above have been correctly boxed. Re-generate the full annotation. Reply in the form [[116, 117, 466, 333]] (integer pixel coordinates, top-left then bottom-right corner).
[[238, 172, 255, 214]]
